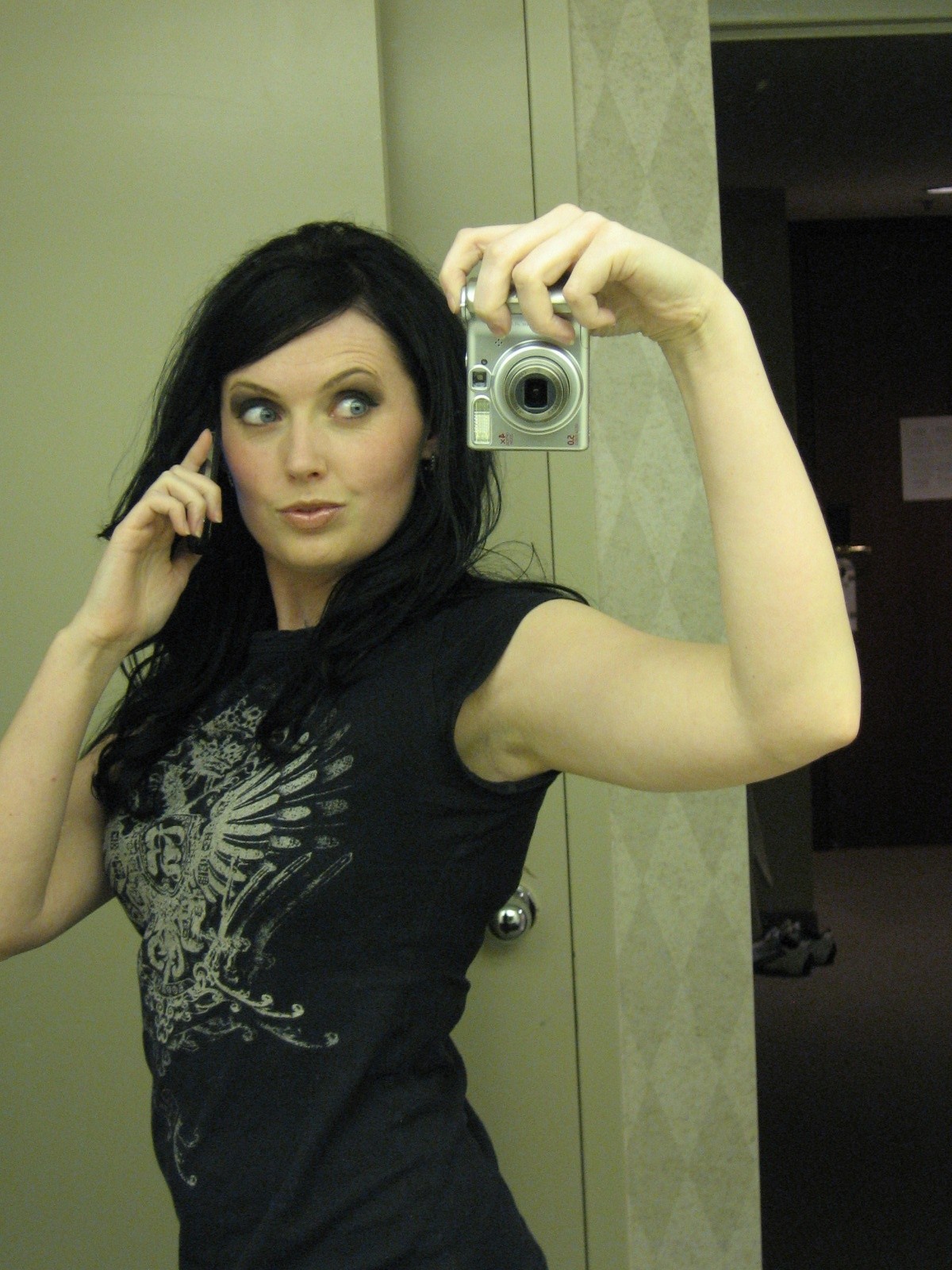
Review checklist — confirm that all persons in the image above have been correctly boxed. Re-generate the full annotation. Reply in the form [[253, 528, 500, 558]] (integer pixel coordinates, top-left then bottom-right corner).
[[0, 203, 862, 1270]]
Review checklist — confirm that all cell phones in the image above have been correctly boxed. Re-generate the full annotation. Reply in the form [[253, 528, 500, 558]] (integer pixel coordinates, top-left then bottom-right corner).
[[184, 427, 223, 557]]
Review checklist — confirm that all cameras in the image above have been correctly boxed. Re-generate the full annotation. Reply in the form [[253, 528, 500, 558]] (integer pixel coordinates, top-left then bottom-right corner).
[[457, 276, 592, 451]]
